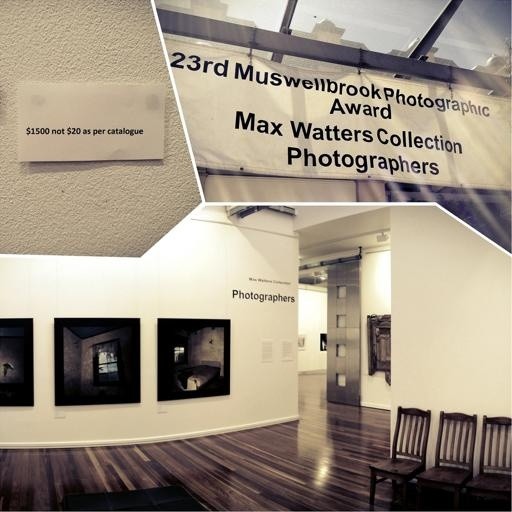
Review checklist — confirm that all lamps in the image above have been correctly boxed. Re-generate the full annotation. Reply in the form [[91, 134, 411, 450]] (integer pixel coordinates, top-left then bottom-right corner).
[[377, 232, 389, 242]]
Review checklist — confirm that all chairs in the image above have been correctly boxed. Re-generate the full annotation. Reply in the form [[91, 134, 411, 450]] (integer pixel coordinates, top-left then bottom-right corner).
[[466, 416, 511, 511], [416, 412, 477, 510], [370, 408, 431, 505]]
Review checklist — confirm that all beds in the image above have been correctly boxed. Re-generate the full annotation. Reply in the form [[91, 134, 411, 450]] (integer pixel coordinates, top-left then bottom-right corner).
[[176, 360, 220, 391]]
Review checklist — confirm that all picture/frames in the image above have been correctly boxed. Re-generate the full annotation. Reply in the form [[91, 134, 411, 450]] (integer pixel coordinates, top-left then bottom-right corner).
[[0, 318, 34, 406], [54, 317, 141, 406], [157, 318, 230, 401]]
